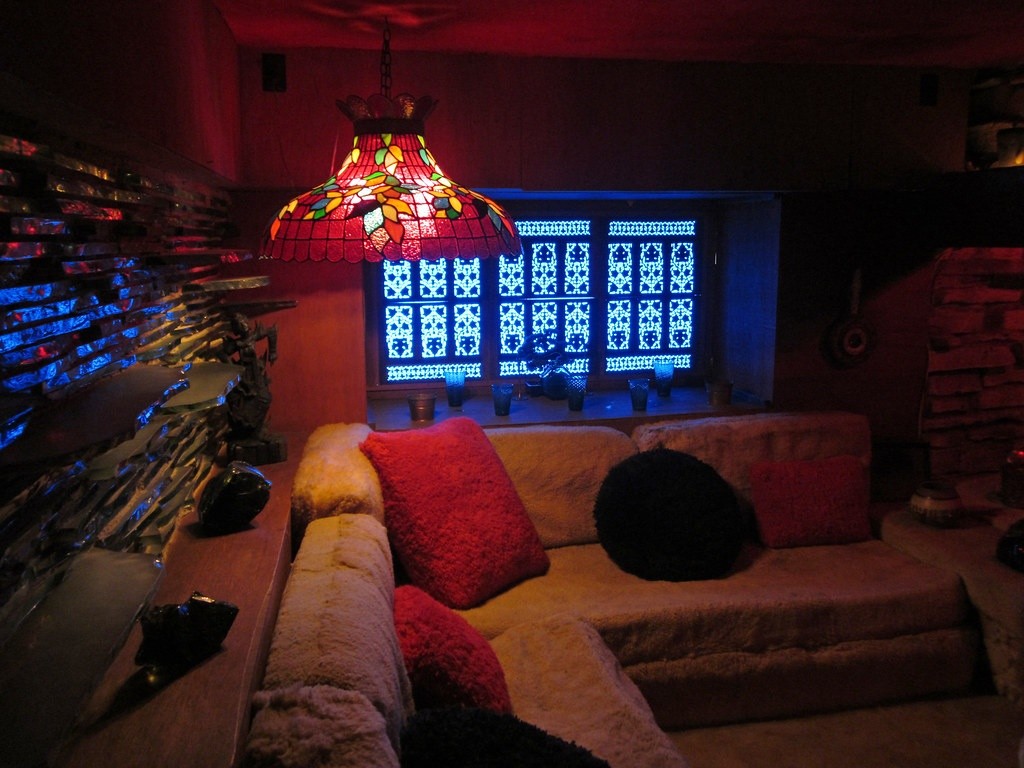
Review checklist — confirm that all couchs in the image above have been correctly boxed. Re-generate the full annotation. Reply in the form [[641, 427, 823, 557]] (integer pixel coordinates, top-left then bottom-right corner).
[[242, 410, 982, 768]]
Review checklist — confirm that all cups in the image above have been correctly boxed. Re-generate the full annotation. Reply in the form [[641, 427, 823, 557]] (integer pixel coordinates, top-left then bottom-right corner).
[[491, 384, 513, 415], [443, 372, 467, 407], [653, 364, 674, 396], [407, 393, 437, 422], [628, 378, 651, 411], [565, 374, 588, 411]]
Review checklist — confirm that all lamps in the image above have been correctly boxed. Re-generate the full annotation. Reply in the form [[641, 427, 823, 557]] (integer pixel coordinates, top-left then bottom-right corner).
[[256, 13, 525, 264]]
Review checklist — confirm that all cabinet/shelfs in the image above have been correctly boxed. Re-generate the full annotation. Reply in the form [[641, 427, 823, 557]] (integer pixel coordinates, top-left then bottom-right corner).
[[0, 80, 298, 768]]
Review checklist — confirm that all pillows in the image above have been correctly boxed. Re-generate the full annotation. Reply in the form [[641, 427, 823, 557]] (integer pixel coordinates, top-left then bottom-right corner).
[[750, 455, 872, 547], [398, 626, 612, 768], [391, 585, 514, 714], [592, 448, 743, 581], [359, 415, 550, 608]]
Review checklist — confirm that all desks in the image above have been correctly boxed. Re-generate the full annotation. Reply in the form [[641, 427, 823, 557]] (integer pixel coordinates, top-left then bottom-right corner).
[[885, 502, 1024, 710]]
